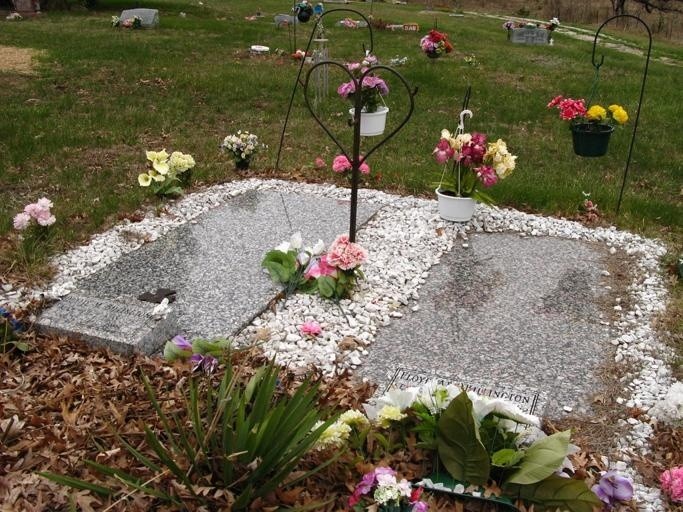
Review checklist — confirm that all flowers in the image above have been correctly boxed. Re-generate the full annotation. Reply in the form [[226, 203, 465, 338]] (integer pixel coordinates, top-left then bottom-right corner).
[[431, 128, 518, 196], [276, 231, 365, 323], [222, 129, 259, 158], [337, 53, 389, 113], [13, 197, 56, 228], [138, 149, 196, 187], [504, 18, 560, 29], [420, 30, 452, 52], [331, 154, 369, 182], [550, 94, 629, 128]]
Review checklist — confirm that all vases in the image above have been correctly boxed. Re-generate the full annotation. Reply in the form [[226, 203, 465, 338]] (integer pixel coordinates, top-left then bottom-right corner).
[[28, 224, 50, 240], [235, 153, 251, 170], [571, 123, 615, 157], [348, 104, 389, 137], [427, 48, 440, 59], [436, 185, 476, 223], [165, 172, 192, 197], [510, 26, 551, 46]]
